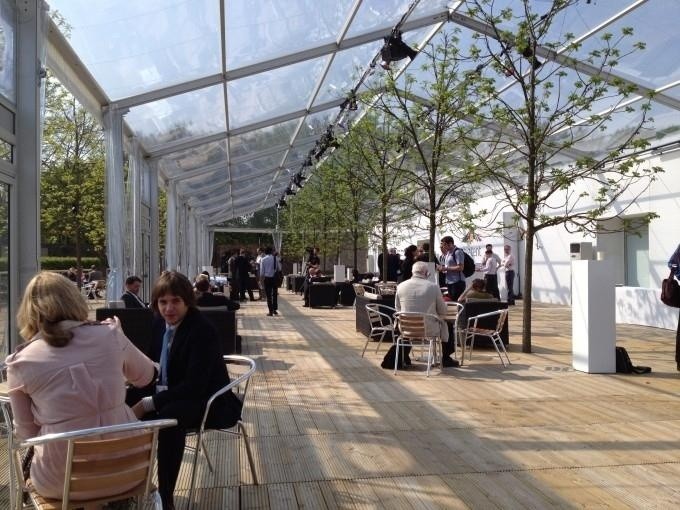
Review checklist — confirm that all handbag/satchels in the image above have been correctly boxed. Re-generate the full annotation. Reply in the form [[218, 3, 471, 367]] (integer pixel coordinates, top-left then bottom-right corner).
[[273, 271, 284, 287], [616, 347, 634, 374], [661, 279, 680, 308]]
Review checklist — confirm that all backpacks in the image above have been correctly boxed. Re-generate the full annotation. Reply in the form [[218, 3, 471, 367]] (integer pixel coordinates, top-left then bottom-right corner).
[[452, 248, 475, 278]]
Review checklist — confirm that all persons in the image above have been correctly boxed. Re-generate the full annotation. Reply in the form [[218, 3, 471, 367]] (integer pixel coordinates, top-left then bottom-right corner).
[[68, 265, 102, 302], [4, 270, 161, 504], [378, 235, 515, 369], [120, 275, 148, 308], [125, 270, 240, 510], [301, 247, 357, 307], [194, 270, 212, 294], [194, 274, 239, 310], [668, 242, 680, 371], [224, 246, 283, 316]]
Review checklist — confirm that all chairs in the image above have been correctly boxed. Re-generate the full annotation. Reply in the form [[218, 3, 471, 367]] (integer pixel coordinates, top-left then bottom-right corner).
[[286, 272, 512, 378], [0, 276, 259, 510]]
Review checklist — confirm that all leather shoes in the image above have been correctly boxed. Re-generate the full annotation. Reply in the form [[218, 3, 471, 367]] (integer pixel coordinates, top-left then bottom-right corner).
[[443, 358, 459, 367], [267, 311, 278, 316]]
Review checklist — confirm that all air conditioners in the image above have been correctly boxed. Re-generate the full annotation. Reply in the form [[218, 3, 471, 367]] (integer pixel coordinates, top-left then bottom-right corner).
[[569, 241, 593, 305]]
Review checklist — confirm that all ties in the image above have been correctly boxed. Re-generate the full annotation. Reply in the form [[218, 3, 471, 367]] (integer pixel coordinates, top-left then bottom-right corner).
[[161, 329, 173, 385]]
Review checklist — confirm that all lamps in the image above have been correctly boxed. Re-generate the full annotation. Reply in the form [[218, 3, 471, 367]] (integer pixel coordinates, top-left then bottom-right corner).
[[340, 90, 359, 113], [276, 131, 340, 207], [380, 30, 416, 62]]
